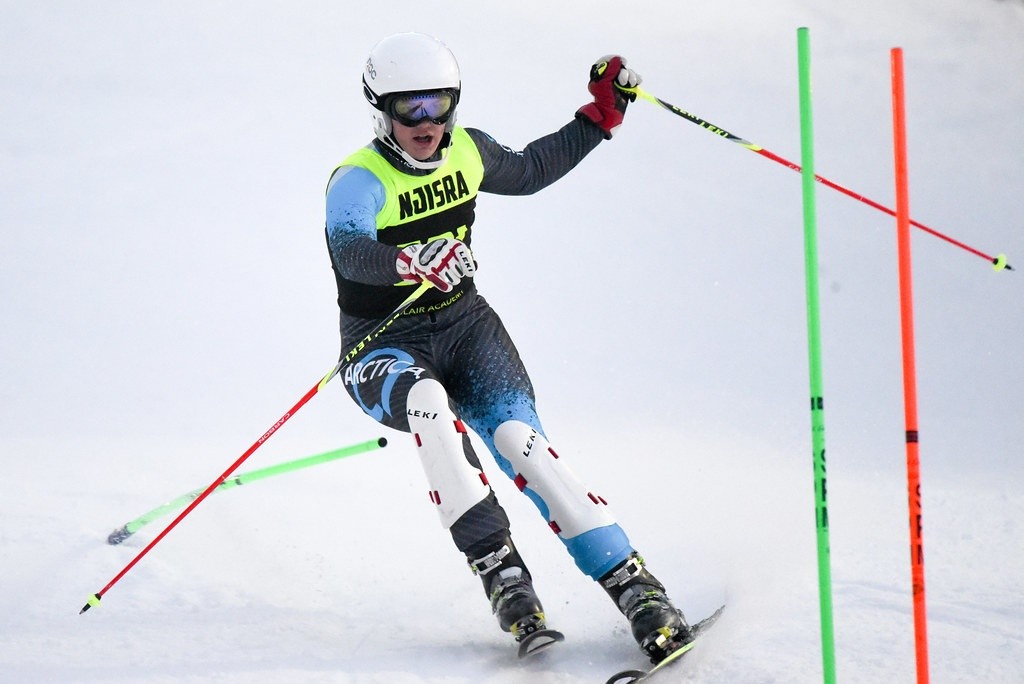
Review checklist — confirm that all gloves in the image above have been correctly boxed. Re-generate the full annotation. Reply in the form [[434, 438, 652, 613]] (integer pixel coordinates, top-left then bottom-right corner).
[[395, 242, 476, 293], [575, 53, 642, 142]]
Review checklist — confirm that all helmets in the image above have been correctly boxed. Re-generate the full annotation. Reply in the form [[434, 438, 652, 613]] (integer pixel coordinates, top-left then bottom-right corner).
[[360, 33, 462, 138]]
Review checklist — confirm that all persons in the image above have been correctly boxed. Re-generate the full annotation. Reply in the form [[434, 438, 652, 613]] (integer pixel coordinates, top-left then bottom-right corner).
[[323, 32, 688, 665]]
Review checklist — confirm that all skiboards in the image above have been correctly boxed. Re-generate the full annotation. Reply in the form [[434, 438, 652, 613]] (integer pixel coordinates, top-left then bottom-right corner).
[[517, 627, 566, 663], [605, 602, 726, 684]]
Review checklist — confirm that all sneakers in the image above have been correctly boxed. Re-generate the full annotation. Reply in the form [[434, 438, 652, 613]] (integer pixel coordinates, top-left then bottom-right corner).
[[468, 549, 543, 635], [603, 562, 683, 655]]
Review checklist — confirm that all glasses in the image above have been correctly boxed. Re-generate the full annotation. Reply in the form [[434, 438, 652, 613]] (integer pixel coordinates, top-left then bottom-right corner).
[[361, 71, 461, 129]]
